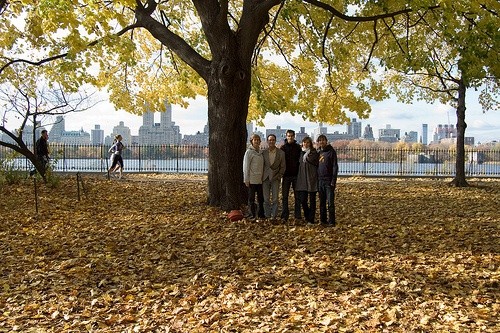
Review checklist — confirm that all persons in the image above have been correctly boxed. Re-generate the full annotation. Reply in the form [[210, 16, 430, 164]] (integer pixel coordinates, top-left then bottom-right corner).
[[105, 134, 127, 179], [313, 135, 339, 226], [242, 134, 265, 220], [279, 130, 303, 219], [29, 130, 49, 178], [262, 134, 286, 221], [293, 137, 320, 224]]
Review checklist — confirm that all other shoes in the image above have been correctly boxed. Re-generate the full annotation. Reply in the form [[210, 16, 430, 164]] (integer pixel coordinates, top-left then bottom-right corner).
[[119, 176, 126, 179], [329, 224, 334, 226], [320, 224, 325, 226], [30, 171, 34, 177]]
